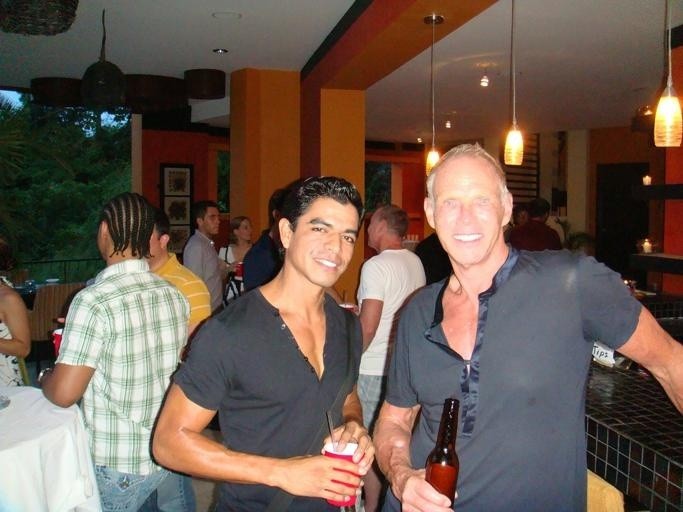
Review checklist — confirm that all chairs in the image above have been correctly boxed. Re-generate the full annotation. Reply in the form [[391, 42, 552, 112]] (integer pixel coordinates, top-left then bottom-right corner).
[[26, 280, 88, 379]]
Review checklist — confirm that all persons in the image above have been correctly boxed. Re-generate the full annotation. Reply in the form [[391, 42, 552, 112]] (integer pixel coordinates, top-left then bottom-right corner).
[[145, 211, 215, 337], [412, 228, 452, 287], [512, 202, 529, 224], [32, 192, 194, 512], [509, 196, 562, 251], [182, 200, 224, 317], [1, 243, 34, 387], [344, 201, 432, 432], [369, 141, 683, 512], [217, 216, 255, 302], [148, 174, 377, 511], [241, 186, 287, 293]]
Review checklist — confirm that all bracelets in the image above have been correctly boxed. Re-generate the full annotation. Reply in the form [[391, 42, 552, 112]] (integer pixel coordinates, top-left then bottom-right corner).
[[36, 366, 53, 382]]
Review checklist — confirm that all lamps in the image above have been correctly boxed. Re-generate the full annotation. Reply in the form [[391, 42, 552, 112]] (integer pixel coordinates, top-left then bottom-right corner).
[[82, 8, 126, 108], [423, 15, 444, 176], [651, 1, 681, 150], [479, 67, 490, 87], [503, 0, 525, 168]]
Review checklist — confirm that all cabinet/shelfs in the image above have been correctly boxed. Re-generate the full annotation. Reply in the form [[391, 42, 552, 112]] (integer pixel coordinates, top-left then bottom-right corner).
[[629, 115, 683, 277]]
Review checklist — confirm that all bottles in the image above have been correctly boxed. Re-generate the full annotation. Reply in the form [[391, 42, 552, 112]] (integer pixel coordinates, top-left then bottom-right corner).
[[424, 397, 459, 509]]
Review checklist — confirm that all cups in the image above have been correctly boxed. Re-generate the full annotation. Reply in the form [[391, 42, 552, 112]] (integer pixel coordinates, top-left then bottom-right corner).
[[338, 303, 354, 311], [234, 261, 244, 280], [321, 441, 364, 508], [52, 329, 63, 356]]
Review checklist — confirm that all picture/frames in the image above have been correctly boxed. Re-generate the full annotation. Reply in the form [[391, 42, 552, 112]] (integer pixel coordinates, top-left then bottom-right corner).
[[159, 160, 195, 264]]
